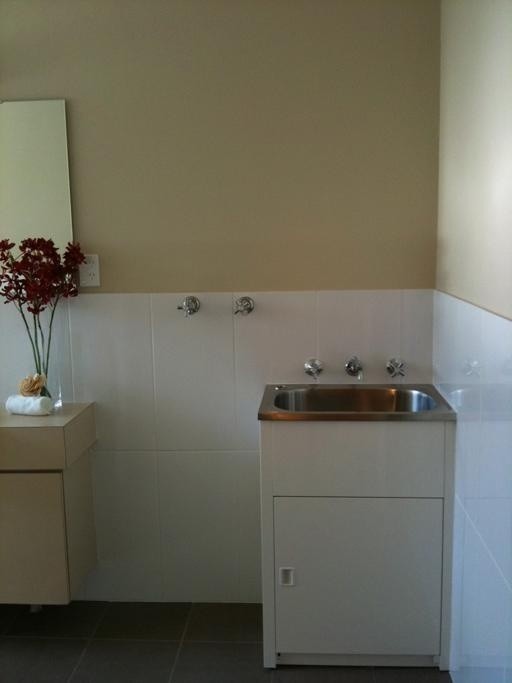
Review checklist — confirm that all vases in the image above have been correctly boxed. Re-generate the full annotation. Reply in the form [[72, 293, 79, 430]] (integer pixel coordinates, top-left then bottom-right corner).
[[37, 373, 62, 409]]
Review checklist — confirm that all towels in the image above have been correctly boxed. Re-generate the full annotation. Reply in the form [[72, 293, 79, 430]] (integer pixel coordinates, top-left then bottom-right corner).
[[5, 395, 53, 415]]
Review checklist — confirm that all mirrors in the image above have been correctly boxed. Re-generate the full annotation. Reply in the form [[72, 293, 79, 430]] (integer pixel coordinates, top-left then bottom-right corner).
[[0, 98, 75, 285]]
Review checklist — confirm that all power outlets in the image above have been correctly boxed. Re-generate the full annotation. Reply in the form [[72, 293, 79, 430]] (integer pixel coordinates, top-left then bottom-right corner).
[[79, 255, 101, 288]]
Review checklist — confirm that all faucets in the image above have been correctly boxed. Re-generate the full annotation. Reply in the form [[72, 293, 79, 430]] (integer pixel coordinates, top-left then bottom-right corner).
[[345, 356, 365, 382]]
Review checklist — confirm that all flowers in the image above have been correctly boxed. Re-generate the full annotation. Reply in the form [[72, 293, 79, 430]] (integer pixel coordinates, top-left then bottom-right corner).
[[0, 238, 85, 397]]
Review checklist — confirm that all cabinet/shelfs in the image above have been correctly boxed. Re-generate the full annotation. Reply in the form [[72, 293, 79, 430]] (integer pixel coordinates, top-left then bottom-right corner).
[[260, 421, 456, 672], [0, 402, 99, 613]]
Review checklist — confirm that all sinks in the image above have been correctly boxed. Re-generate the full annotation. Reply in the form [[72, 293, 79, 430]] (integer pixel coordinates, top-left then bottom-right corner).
[[258, 381, 458, 422]]
